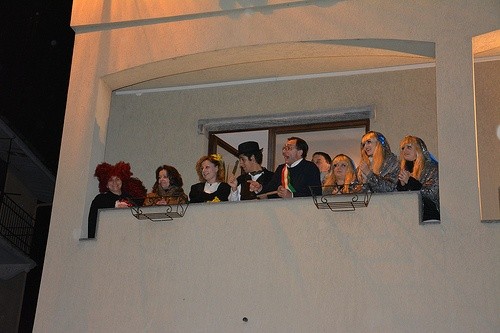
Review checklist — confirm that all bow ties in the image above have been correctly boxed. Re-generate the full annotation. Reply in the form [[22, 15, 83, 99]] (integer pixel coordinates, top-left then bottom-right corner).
[[249, 170, 264, 176]]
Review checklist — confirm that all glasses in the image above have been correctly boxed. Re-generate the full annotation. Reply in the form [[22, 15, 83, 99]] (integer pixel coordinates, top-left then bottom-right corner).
[[282, 146, 296, 151]]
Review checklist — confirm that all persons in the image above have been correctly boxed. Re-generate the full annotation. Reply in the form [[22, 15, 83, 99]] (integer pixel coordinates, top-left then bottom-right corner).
[[143, 164, 189, 206], [227, 140, 275, 202], [395, 134, 440, 222], [189, 153, 233, 205], [318, 153, 364, 196], [84, 161, 141, 238], [245, 136, 323, 199], [311, 152, 331, 180], [352, 130, 400, 193]]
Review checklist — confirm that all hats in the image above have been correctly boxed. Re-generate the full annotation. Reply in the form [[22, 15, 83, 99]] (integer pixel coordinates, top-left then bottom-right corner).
[[235, 142, 263, 155]]
